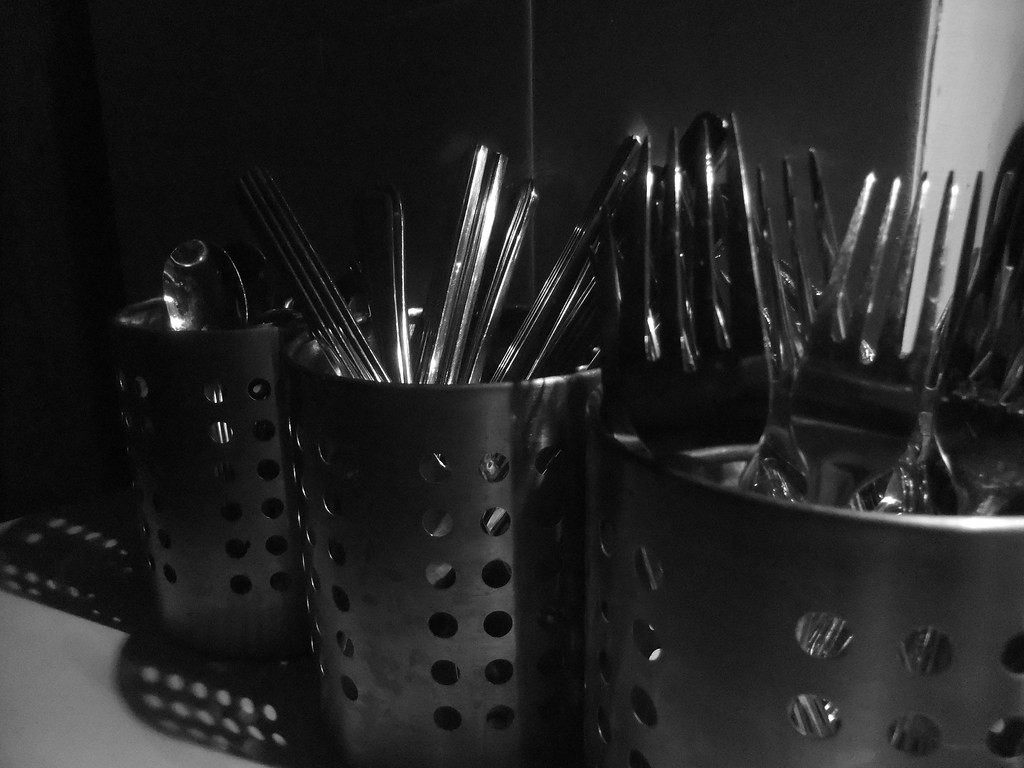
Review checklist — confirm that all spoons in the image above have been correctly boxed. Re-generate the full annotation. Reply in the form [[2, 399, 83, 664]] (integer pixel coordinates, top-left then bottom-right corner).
[[158, 240, 296, 330]]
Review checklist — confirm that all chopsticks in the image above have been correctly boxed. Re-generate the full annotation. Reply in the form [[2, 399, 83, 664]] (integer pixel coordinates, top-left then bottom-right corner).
[[232, 133, 642, 381]]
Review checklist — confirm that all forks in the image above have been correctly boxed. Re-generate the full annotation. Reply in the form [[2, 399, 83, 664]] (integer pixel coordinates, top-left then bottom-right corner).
[[601, 115, 1024, 520]]
